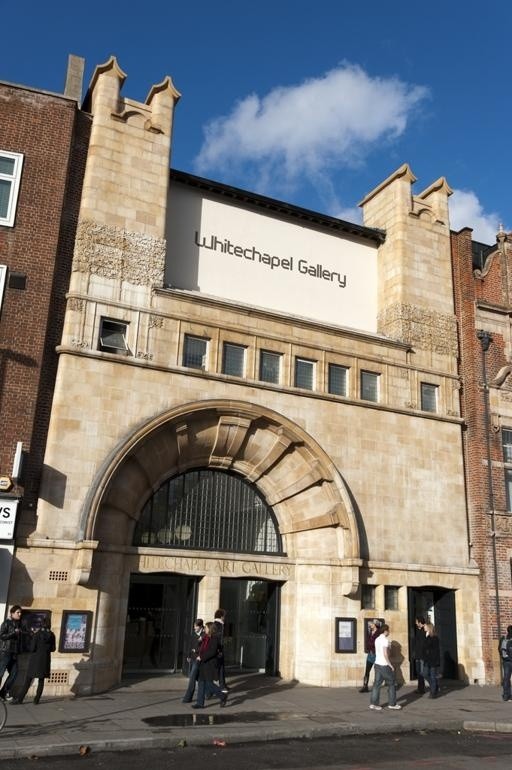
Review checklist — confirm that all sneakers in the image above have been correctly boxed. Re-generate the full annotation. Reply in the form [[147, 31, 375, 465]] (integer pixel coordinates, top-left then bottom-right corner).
[[369, 705, 382, 710], [388, 704, 401, 709]]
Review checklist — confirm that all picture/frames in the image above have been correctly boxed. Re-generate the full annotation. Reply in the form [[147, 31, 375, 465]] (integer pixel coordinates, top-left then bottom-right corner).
[[335, 618, 357, 654], [58, 609, 93, 653], [364, 618, 385, 653]]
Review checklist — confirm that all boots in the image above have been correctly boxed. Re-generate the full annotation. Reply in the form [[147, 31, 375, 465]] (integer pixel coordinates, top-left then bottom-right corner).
[[360, 677, 368, 692]]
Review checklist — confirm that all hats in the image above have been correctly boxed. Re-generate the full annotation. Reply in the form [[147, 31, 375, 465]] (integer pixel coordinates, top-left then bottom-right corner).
[[368, 619, 380, 626]]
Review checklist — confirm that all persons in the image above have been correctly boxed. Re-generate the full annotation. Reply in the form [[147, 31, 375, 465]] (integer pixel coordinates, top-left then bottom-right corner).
[[0, 603, 29, 702], [190, 622, 228, 708], [497, 625, 511, 699], [420, 622, 441, 698], [412, 616, 440, 693], [14, 616, 56, 704], [368, 624, 402, 710], [359, 618, 397, 693], [211, 608, 233, 693], [182, 619, 213, 702]]
